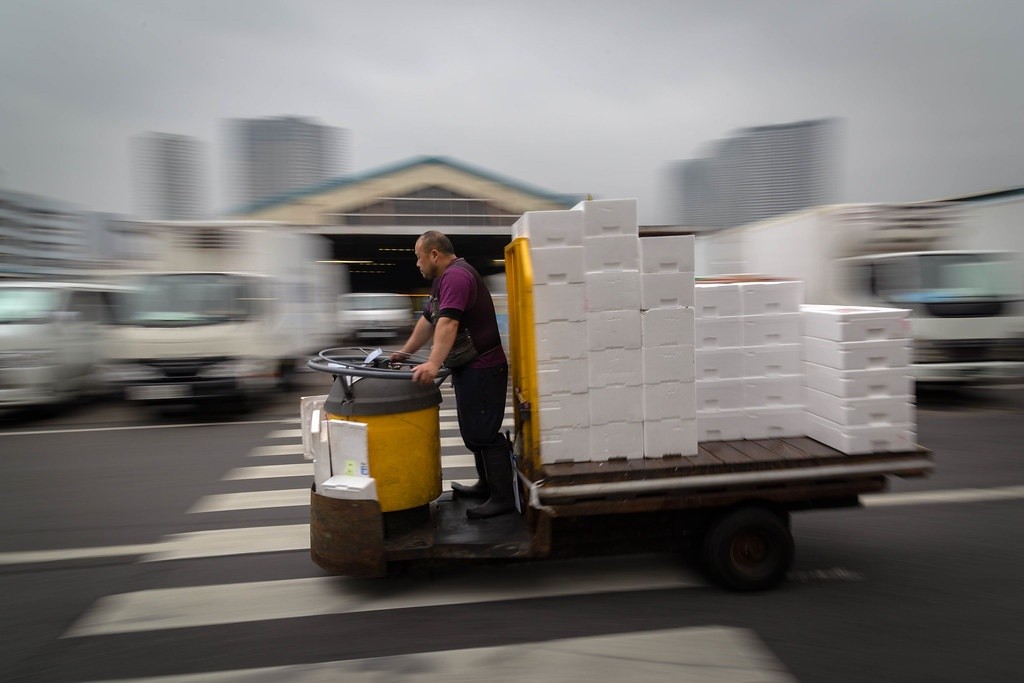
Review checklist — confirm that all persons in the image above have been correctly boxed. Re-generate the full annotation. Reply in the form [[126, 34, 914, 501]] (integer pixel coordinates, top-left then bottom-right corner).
[[389, 230, 517, 520]]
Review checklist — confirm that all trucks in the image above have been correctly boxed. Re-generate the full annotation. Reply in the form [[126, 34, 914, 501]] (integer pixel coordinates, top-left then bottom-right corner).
[[276, 238, 935, 595]]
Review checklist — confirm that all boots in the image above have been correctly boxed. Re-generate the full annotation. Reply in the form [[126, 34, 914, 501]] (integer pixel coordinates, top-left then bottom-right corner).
[[452, 433, 503, 498], [466, 446, 513, 519]]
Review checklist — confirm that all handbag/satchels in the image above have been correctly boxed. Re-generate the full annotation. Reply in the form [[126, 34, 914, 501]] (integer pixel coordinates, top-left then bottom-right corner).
[[442, 328, 479, 368]]
